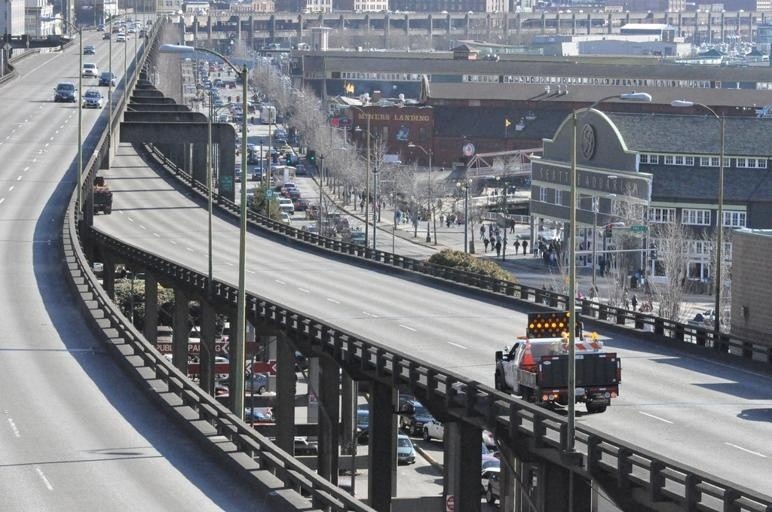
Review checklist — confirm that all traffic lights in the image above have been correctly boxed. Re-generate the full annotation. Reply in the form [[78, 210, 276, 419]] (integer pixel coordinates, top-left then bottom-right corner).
[[309, 150, 315, 163], [287, 154, 292, 165]]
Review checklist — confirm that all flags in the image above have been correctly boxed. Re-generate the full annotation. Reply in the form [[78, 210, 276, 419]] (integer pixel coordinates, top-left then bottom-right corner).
[[505, 119, 511, 127], [342, 79, 354, 94]]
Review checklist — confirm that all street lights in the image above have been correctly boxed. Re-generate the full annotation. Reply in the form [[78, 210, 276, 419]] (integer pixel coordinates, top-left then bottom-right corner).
[[81, 5, 111, 136], [671, 98, 724, 349], [39, 17, 83, 211], [409, 144, 432, 243], [356, 128, 378, 223], [158, 44, 248, 421], [567, 92, 652, 445], [337, 103, 370, 248]]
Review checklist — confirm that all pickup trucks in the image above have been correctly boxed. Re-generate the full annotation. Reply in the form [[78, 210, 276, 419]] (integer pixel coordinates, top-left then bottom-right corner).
[[494, 335, 621, 414]]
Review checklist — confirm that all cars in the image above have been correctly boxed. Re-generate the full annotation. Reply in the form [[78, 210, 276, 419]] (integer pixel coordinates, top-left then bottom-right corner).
[[247, 143, 258, 165], [83, 91, 103, 109], [198, 58, 262, 124], [236, 169, 243, 182], [213, 371, 269, 396], [271, 174, 370, 247], [265, 129, 293, 163], [54, 83, 77, 103], [270, 438, 317, 454], [399, 394, 538, 510], [252, 167, 266, 179], [82, 63, 98, 78], [99, 72, 115, 86], [355, 403, 415, 465], [246, 408, 265, 422], [97, 16, 153, 42], [83, 46, 94, 54]]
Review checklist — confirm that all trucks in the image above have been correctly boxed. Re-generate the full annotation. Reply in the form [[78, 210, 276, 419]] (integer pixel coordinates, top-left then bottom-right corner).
[[261, 106, 277, 125]]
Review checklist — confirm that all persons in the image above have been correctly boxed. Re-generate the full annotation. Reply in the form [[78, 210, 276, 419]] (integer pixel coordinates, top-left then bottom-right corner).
[[622, 269, 653, 312], [342, 185, 611, 277], [440, 167, 444, 171], [542, 284, 598, 299]]
